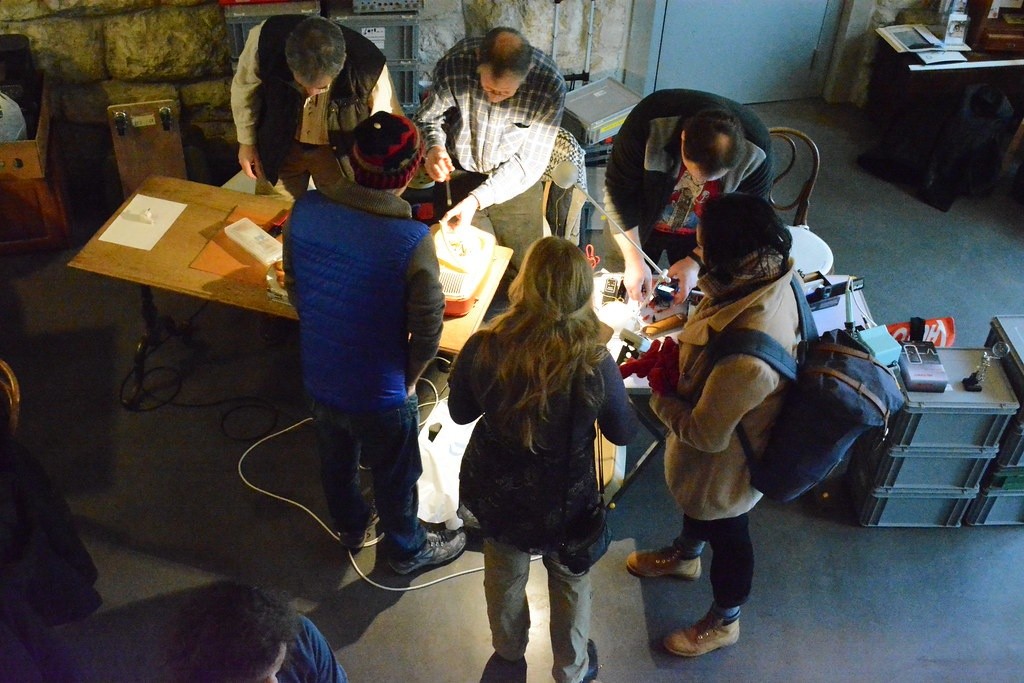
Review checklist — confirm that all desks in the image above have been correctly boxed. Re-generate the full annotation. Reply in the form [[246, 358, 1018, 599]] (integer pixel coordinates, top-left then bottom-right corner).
[[587, 274, 669, 513], [67, 173, 513, 408]]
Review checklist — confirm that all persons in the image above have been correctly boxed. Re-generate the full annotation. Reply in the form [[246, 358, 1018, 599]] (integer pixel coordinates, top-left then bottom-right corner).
[[282, 112, 469, 578], [413, 26, 566, 289], [445, 239, 641, 683], [170, 585, 349, 683], [229, 14, 391, 202], [627, 192, 804, 658], [607, 88, 775, 310]]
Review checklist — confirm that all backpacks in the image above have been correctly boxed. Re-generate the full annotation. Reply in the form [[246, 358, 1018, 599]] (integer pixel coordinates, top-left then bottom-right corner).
[[693, 272, 906, 506]]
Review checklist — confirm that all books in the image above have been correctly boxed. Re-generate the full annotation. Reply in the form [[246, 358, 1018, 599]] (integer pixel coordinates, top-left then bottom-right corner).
[[874, 22, 972, 53]]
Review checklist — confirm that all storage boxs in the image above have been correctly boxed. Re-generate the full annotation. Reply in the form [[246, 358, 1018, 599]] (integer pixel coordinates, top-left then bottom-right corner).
[[220, 0, 425, 122], [576, 140, 614, 167], [0, 69, 68, 261], [841, 313, 1024, 531], [560, 75, 645, 146]]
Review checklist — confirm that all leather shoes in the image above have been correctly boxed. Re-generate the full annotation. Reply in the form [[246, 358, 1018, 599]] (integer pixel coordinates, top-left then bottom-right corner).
[[582, 638, 599, 683]]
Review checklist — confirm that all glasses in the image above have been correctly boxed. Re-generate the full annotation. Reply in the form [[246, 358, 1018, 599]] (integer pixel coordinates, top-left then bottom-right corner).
[[479, 84, 517, 97]]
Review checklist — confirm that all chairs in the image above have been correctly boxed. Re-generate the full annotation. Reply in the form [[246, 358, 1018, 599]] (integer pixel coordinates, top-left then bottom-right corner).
[[766, 126, 820, 231]]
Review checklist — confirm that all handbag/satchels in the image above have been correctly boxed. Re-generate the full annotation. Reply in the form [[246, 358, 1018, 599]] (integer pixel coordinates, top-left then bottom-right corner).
[[558, 495, 612, 575]]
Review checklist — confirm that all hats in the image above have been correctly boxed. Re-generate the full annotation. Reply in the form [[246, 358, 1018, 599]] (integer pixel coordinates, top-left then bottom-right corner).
[[347, 111, 425, 189]]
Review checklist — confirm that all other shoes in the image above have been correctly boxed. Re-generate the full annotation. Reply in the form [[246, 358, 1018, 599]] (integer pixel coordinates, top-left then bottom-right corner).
[[339, 530, 368, 549], [389, 528, 467, 577], [256, 312, 287, 345], [661, 605, 741, 658], [625, 540, 702, 581]]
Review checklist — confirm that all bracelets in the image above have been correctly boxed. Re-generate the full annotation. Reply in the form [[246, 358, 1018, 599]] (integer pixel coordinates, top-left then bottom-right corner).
[[686, 251, 704, 268]]
